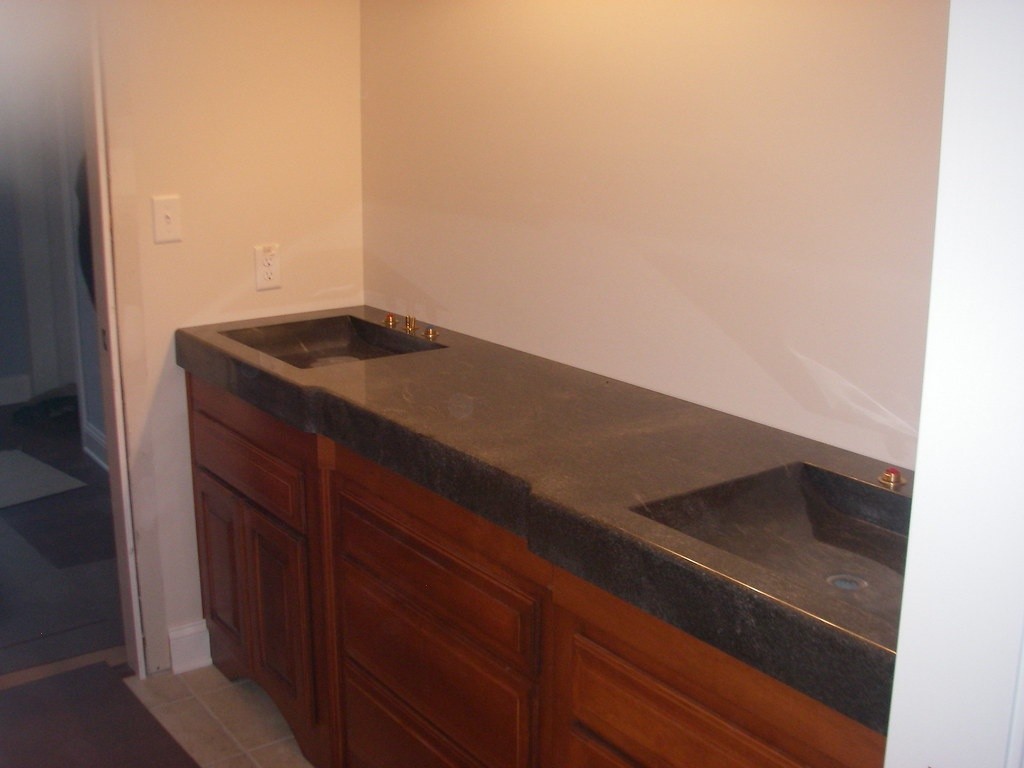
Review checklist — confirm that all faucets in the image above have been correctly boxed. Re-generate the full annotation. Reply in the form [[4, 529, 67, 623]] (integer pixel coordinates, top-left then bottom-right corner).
[[405, 315, 416, 329]]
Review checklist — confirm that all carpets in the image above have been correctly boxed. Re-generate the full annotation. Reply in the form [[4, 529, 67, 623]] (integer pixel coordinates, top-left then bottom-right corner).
[[0, 659, 201, 768], [0, 483, 115, 569]]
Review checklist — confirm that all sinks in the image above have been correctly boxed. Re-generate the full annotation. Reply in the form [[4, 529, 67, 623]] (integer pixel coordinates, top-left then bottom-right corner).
[[627, 460, 914, 631], [216, 314, 451, 370]]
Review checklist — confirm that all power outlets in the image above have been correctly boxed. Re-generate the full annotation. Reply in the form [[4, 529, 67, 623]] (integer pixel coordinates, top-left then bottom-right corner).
[[254, 243, 280, 289]]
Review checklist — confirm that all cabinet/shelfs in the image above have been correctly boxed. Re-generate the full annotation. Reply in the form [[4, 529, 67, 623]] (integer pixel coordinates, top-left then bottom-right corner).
[[183, 372, 887, 768]]
